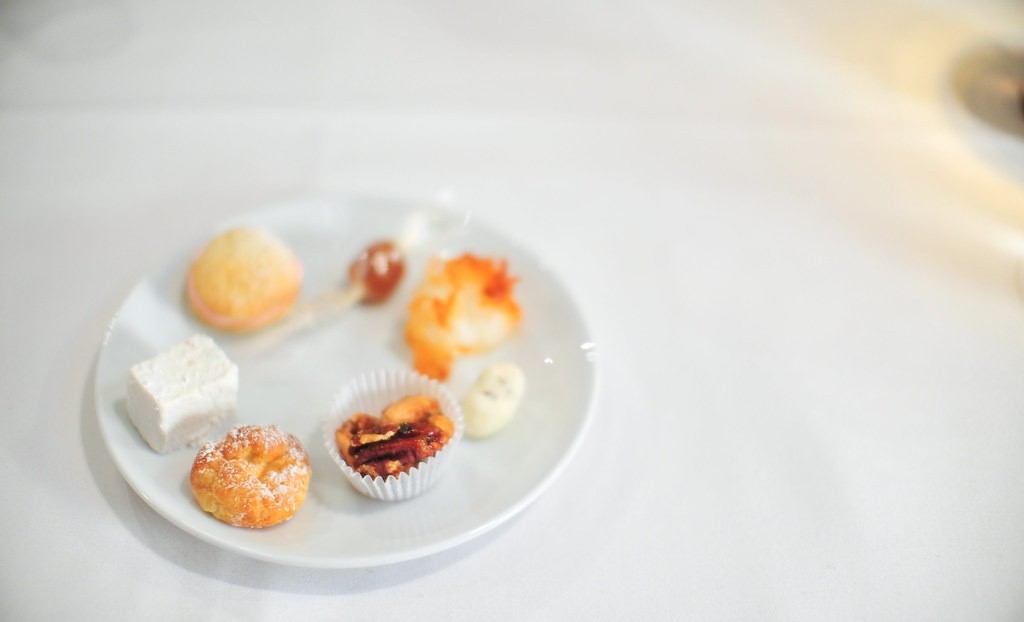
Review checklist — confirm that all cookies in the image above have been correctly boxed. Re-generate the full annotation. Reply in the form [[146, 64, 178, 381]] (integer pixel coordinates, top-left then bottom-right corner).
[[336, 394, 455, 481]]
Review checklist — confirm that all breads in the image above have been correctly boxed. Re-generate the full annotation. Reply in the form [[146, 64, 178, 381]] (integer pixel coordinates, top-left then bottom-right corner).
[[185, 226, 305, 332], [190, 424, 314, 529]]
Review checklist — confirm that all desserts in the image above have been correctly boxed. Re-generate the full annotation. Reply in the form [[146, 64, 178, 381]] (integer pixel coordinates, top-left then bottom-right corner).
[[126, 333, 241, 456], [462, 362, 527, 440]]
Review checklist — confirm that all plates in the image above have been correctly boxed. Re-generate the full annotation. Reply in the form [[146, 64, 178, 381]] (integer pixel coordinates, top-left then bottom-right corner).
[[92, 195, 599, 568]]
